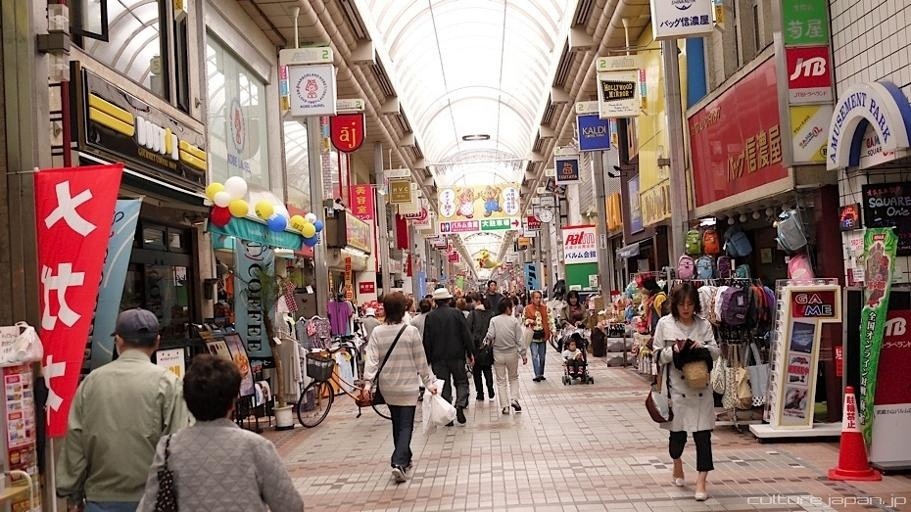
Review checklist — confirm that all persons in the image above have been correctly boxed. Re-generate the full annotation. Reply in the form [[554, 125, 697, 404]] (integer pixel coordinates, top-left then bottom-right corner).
[[136, 353, 303, 512], [55, 308, 190, 512], [642, 278, 667, 335], [362, 281, 589, 483], [652, 283, 719, 502]]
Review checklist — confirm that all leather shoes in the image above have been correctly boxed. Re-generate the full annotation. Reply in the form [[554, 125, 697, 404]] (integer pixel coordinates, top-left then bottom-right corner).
[[693, 490, 709, 502], [672, 469, 687, 486]]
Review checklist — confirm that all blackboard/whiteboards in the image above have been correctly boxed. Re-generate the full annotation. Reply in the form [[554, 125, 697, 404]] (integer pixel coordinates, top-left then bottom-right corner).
[[862, 181, 911, 257]]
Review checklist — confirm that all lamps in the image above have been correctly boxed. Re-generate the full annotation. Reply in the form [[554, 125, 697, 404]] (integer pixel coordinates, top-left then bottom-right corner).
[[725, 204, 774, 226]]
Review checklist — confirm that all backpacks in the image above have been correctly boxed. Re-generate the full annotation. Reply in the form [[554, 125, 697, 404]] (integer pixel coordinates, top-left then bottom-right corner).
[[698, 278, 776, 331], [775, 206, 809, 252], [685, 230, 701, 254], [718, 255, 733, 279], [725, 227, 752, 257], [695, 256, 714, 279], [786, 254, 814, 283], [703, 228, 720, 255], [679, 254, 694, 279]]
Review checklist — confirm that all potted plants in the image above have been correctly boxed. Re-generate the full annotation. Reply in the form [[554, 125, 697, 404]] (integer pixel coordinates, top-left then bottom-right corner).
[[257, 262, 295, 428]]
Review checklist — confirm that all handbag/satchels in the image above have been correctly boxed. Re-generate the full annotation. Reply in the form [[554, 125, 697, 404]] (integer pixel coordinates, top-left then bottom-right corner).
[[532, 327, 547, 340], [372, 378, 387, 406], [714, 343, 771, 410], [523, 324, 535, 350], [477, 338, 494, 369], [149, 432, 178, 512], [646, 391, 674, 424], [675, 348, 714, 389]]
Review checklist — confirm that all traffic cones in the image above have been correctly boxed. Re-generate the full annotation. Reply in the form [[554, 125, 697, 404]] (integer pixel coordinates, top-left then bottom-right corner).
[[826, 384, 885, 482]]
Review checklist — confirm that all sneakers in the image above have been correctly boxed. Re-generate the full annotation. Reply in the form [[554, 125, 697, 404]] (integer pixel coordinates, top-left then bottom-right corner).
[[511, 399, 522, 412], [533, 376, 546, 383], [501, 406, 509, 414], [488, 388, 495, 398], [455, 405, 466, 424], [476, 393, 484, 401], [392, 463, 412, 484]]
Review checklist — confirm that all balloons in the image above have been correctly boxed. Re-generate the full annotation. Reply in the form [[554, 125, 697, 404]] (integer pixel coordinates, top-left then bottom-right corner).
[[205, 176, 322, 246]]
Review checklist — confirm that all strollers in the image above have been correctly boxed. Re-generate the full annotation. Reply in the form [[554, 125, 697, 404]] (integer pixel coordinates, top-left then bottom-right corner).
[[558, 322, 596, 387]]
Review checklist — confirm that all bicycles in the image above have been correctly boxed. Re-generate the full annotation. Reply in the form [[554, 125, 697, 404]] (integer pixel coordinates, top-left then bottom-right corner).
[[292, 333, 391, 429]]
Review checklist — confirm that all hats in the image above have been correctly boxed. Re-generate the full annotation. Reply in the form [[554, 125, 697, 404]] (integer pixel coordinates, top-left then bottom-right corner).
[[110, 307, 161, 342], [430, 287, 454, 302]]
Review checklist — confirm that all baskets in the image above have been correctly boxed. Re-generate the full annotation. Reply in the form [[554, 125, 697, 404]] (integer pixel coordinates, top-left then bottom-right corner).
[[305, 352, 335, 383]]
